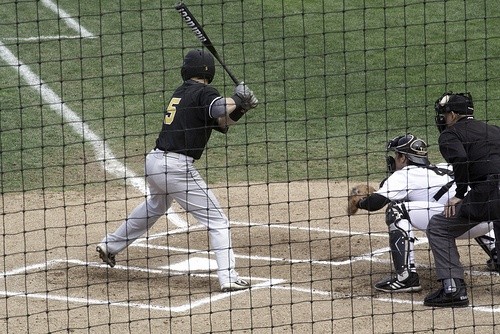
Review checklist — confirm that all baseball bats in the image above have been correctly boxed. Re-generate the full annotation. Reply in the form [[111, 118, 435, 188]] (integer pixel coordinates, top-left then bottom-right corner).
[[174, 1, 240, 87]]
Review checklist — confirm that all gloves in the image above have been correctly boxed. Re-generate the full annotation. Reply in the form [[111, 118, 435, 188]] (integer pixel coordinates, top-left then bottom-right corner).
[[235, 81, 254, 101], [240, 91, 259, 111]]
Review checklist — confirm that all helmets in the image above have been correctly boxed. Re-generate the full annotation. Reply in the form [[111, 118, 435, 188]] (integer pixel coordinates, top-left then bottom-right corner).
[[181, 49, 215, 84], [387, 132, 432, 165], [434, 90, 474, 117]]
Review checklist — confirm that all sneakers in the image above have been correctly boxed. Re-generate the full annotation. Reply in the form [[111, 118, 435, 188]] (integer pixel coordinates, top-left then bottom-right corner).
[[424, 278, 470, 308], [220, 276, 252, 291], [373, 270, 423, 293], [96, 242, 116, 268], [487, 259, 500, 272]]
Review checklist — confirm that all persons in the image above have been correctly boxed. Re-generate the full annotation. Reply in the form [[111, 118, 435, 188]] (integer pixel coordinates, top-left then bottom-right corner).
[[423, 91, 500, 309], [355, 132, 500, 292], [97, 50, 258, 291]]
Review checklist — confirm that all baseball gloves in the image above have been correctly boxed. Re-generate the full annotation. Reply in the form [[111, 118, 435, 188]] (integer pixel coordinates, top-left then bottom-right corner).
[[347, 185, 377, 216]]
